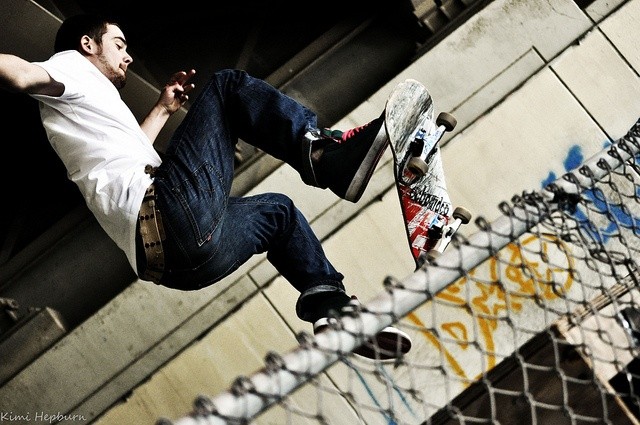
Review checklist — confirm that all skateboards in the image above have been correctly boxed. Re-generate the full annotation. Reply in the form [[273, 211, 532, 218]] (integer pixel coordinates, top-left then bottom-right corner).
[[384, 78, 472, 273]]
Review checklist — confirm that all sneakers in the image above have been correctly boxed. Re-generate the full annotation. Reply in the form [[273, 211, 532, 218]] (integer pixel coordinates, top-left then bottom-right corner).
[[321, 108, 392, 203], [313, 295, 412, 362]]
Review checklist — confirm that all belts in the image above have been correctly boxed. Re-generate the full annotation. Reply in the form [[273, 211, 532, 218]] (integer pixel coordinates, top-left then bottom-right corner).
[[138, 185, 171, 285]]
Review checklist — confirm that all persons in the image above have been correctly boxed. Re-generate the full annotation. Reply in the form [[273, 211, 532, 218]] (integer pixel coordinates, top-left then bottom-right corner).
[[0, 14, 411, 354]]
